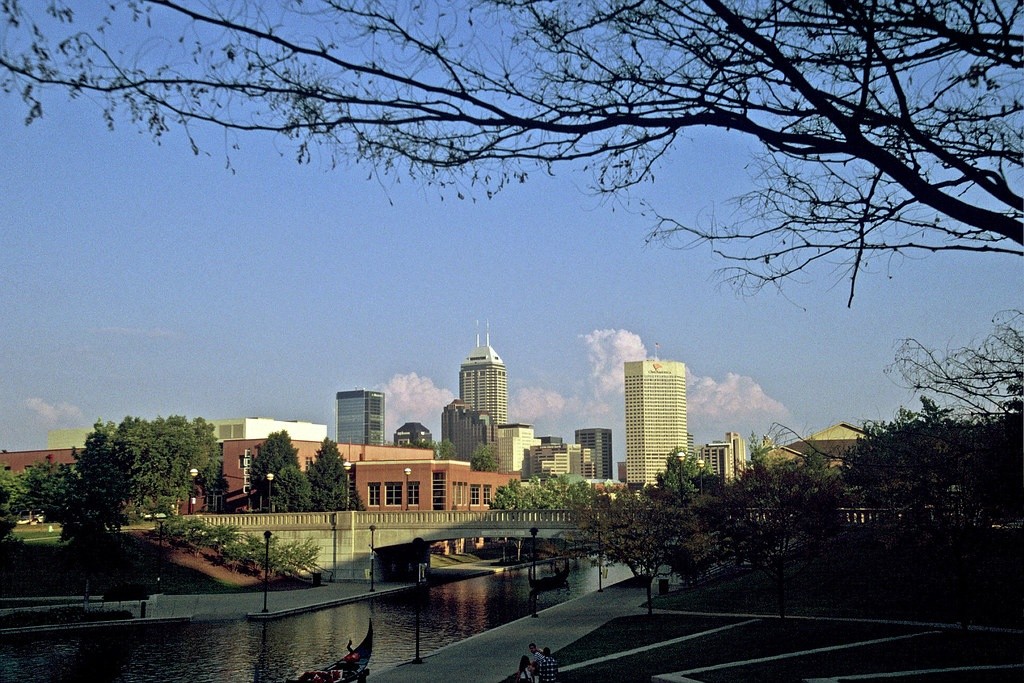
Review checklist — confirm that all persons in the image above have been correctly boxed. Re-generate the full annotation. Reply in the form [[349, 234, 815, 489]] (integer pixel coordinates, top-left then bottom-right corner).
[[516, 643, 558, 683]]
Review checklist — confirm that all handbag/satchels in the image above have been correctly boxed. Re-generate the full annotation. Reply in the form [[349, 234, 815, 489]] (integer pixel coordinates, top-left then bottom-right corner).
[[517, 670, 531, 683]]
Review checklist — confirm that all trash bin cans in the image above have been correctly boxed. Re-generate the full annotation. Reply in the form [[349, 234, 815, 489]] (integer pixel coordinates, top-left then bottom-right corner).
[[658, 578, 669, 594], [312, 572, 322, 587]]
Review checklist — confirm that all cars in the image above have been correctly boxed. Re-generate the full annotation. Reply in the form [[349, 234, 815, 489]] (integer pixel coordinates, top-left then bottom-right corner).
[[141, 509, 167, 522], [15, 508, 47, 525]]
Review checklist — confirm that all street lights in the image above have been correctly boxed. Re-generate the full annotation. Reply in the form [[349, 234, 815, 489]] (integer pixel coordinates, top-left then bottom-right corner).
[[404, 468, 412, 510], [261, 530, 272, 612], [528, 527, 539, 618], [266, 473, 275, 512], [696, 459, 705, 496], [343, 461, 352, 511], [410, 537, 426, 664], [675, 451, 686, 507], [188, 468, 199, 515], [368, 525, 375, 592]]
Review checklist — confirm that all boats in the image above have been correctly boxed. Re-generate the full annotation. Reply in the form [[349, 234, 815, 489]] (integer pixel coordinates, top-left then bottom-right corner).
[[528, 556, 570, 592], [286, 617, 373, 683]]
